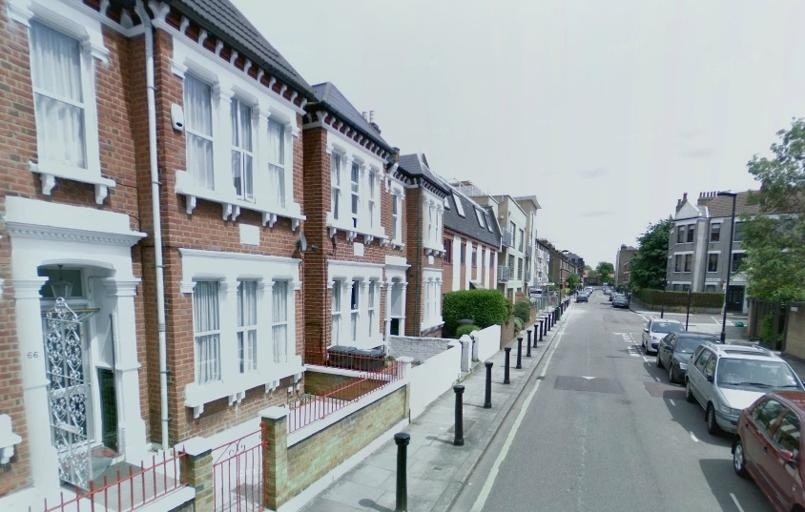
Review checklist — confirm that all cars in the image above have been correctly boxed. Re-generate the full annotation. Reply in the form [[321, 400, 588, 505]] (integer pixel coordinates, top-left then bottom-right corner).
[[576, 285, 594, 304], [642, 316, 684, 354], [602, 283, 628, 310], [730, 389, 805, 512], [529, 288, 543, 299], [656, 330, 719, 382]]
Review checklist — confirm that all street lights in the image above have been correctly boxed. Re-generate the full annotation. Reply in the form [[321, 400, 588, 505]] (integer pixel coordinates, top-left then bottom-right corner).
[[714, 191, 737, 342], [559, 250, 569, 306]]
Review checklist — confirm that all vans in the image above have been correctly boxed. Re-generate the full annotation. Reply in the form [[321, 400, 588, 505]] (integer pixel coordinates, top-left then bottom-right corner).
[[685, 342, 804, 438]]
